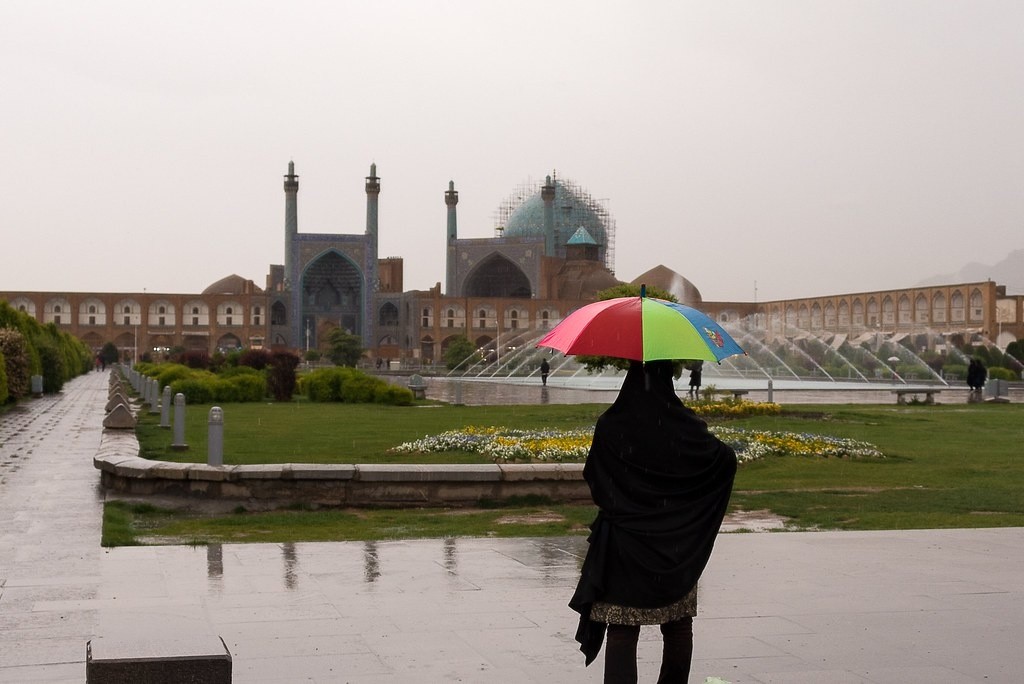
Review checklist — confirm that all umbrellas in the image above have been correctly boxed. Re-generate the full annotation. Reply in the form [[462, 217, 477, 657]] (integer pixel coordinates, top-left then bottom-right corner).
[[535, 283, 748, 368]]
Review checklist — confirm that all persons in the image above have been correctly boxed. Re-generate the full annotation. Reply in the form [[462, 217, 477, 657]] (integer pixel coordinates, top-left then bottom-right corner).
[[96, 355, 101, 372], [568, 360, 738, 684], [101, 355, 106, 372], [540, 358, 550, 386], [966, 359, 987, 393], [688, 364, 701, 393]]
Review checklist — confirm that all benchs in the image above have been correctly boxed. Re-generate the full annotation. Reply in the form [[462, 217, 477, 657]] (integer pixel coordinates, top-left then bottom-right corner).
[[696, 388, 748, 399], [891, 389, 941, 403]]
